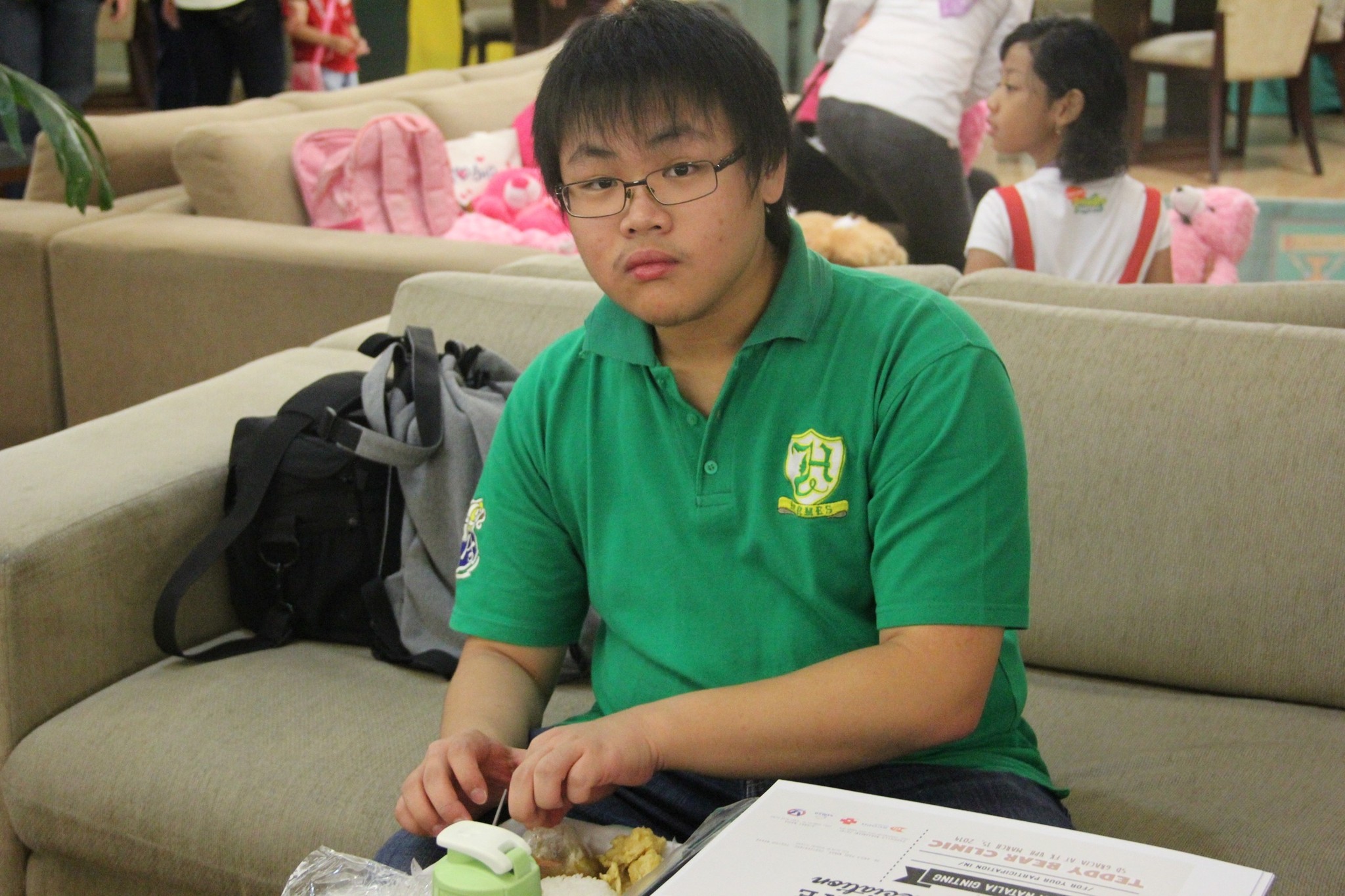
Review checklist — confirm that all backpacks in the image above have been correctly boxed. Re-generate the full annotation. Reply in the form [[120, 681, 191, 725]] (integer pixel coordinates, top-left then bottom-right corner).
[[151, 370, 405, 662], [290, 110, 457, 238], [327, 327, 603, 684]]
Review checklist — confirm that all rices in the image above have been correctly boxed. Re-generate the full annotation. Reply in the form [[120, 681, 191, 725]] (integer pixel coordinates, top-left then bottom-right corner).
[[540, 873, 616, 896]]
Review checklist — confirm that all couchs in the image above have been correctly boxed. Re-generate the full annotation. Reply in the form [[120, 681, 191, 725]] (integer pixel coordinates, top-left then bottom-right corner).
[[1, 272, 1345, 896], [3, 40, 1345, 448]]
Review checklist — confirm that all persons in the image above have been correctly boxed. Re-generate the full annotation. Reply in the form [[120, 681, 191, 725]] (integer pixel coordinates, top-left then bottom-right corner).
[[961, 13, 1171, 294], [149, 0, 285, 114], [365, 4, 1079, 888], [284, 2, 372, 95], [2, 1, 131, 207], [807, 1, 1040, 271]]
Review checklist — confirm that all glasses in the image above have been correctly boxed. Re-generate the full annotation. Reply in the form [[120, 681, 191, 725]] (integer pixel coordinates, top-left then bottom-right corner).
[[554, 138, 750, 218]]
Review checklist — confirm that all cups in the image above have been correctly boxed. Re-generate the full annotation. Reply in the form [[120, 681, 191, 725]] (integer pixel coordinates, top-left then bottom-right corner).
[[428, 820, 542, 896]]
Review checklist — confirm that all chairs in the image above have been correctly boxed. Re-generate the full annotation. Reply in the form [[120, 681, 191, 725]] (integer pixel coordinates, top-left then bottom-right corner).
[[1125, 0, 1344, 184]]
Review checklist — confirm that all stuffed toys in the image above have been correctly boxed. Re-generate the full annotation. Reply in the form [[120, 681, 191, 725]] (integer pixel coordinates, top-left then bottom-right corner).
[[476, 167, 566, 238], [1166, 181, 1260, 290], [794, 208, 914, 271]]
